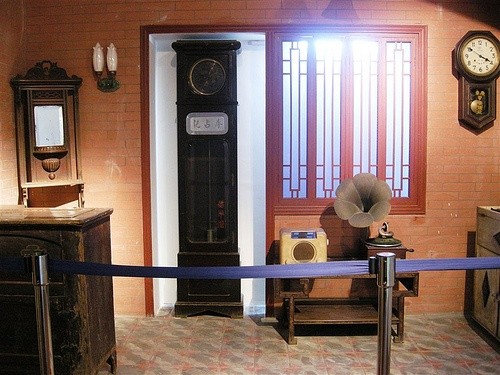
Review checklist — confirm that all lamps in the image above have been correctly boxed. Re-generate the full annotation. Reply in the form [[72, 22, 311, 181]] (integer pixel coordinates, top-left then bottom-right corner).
[[92, 42, 121, 93]]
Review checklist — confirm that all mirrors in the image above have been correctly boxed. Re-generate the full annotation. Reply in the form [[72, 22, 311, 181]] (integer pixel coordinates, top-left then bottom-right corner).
[[10, 61, 85, 208]]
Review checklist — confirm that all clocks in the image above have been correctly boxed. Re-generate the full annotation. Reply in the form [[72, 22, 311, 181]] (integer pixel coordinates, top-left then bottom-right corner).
[[189, 58, 227, 96], [452, 31, 500, 132]]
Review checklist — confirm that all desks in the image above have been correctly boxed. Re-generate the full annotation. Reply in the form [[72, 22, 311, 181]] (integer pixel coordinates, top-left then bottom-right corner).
[[273, 257, 420, 345]]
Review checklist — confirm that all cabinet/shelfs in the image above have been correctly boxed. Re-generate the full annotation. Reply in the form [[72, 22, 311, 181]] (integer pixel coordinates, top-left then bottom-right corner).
[[471, 206, 500, 341], [0, 206, 117, 375]]
